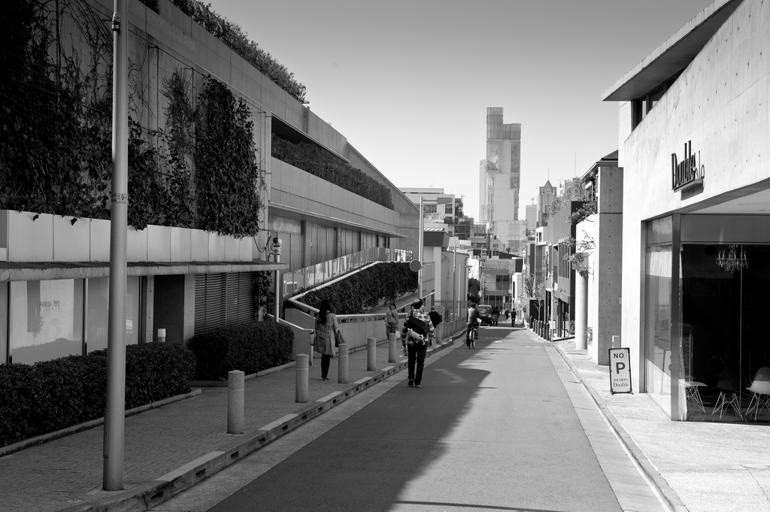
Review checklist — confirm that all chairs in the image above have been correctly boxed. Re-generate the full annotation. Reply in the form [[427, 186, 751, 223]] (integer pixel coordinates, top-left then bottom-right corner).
[[686, 367, 770, 421]]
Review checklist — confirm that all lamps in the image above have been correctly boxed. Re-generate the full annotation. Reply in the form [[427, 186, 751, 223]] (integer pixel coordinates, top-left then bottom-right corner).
[[715, 244, 749, 274]]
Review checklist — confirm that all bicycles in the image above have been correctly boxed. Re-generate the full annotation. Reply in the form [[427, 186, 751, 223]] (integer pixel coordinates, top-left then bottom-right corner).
[[468, 316, 481, 350]]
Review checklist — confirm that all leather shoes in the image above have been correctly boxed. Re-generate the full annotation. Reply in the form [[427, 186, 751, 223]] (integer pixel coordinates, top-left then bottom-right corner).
[[415, 385, 423, 388], [408, 382, 414, 387]]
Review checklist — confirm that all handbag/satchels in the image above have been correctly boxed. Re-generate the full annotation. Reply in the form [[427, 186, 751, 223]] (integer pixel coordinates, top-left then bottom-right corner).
[[408, 316, 427, 336], [334, 330, 345, 348]]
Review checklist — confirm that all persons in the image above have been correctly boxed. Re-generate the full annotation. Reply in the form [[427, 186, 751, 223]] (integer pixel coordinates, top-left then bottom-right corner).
[[402, 299, 435, 388], [384, 302, 398, 339], [465, 303, 479, 345], [313, 299, 340, 382], [511, 308, 516, 327], [490, 302, 509, 327], [428, 306, 442, 344]]
